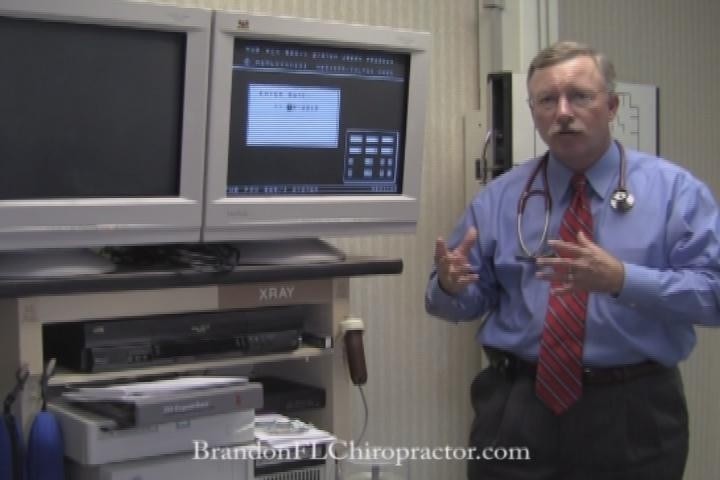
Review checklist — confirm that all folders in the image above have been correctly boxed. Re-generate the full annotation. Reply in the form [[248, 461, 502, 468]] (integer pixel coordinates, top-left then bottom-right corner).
[[63, 373, 266, 430]]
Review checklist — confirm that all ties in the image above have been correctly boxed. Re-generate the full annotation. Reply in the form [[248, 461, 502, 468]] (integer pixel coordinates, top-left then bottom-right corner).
[[535, 173, 595, 415]]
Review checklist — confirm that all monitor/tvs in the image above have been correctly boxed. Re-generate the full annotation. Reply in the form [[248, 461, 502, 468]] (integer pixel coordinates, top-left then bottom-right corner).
[[0, 0, 215, 283], [199, 8, 432, 264]]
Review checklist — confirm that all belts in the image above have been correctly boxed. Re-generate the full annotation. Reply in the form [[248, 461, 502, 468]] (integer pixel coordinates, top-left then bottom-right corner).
[[584, 363, 658, 384]]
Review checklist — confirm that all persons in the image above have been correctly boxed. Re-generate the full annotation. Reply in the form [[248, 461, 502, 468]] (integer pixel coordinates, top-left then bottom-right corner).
[[424, 42, 720, 480]]
